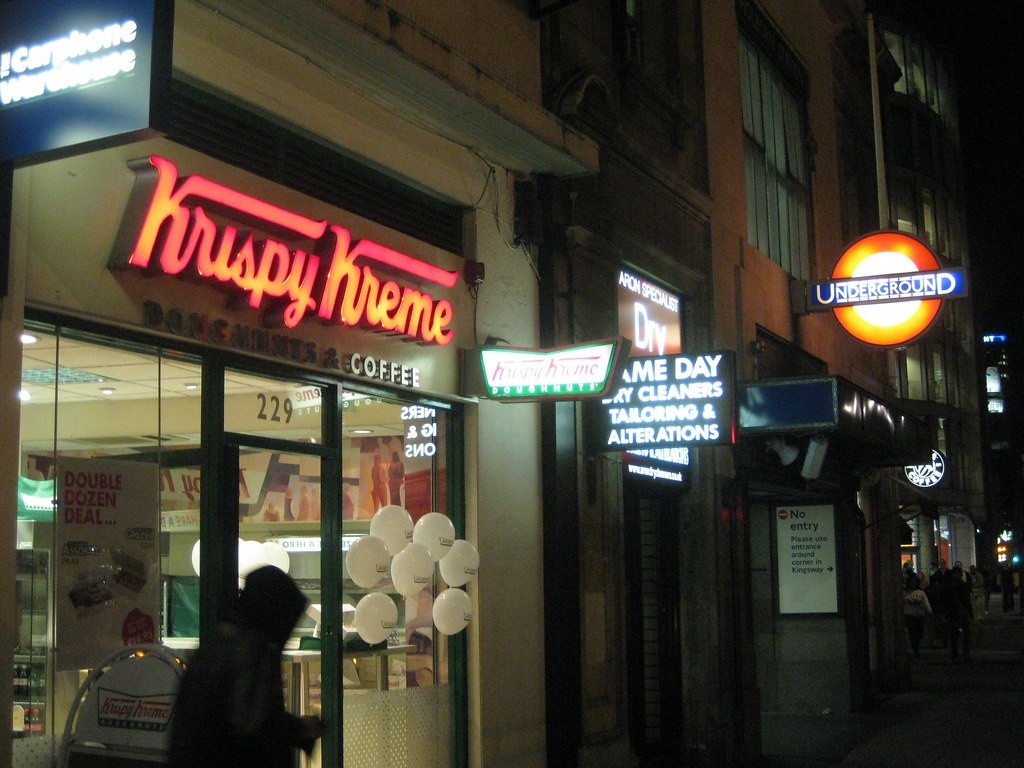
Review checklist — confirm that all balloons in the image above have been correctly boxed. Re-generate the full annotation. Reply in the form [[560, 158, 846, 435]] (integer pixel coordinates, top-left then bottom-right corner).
[[191, 538, 290, 598], [346, 505, 480, 646]]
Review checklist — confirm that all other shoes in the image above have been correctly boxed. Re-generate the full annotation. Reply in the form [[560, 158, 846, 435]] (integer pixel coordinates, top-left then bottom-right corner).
[[985, 610, 992, 614], [914, 650, 920, 657]]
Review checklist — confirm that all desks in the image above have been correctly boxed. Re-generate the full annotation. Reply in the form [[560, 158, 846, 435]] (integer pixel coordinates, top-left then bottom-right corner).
[[163, 643, 418, 768]]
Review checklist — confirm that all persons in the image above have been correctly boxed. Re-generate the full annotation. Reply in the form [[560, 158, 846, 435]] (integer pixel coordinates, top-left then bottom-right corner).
[[168, 565, 325, 768], [902, 561, 1014, 670]]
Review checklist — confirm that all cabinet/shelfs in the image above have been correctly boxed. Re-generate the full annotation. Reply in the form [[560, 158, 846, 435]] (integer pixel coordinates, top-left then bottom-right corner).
[[11, 550, 55, 737]]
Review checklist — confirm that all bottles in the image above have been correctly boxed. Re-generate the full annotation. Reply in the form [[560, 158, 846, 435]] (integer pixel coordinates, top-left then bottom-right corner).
[[13, 664, 46, 697], [14, 709, 46, 738]]
